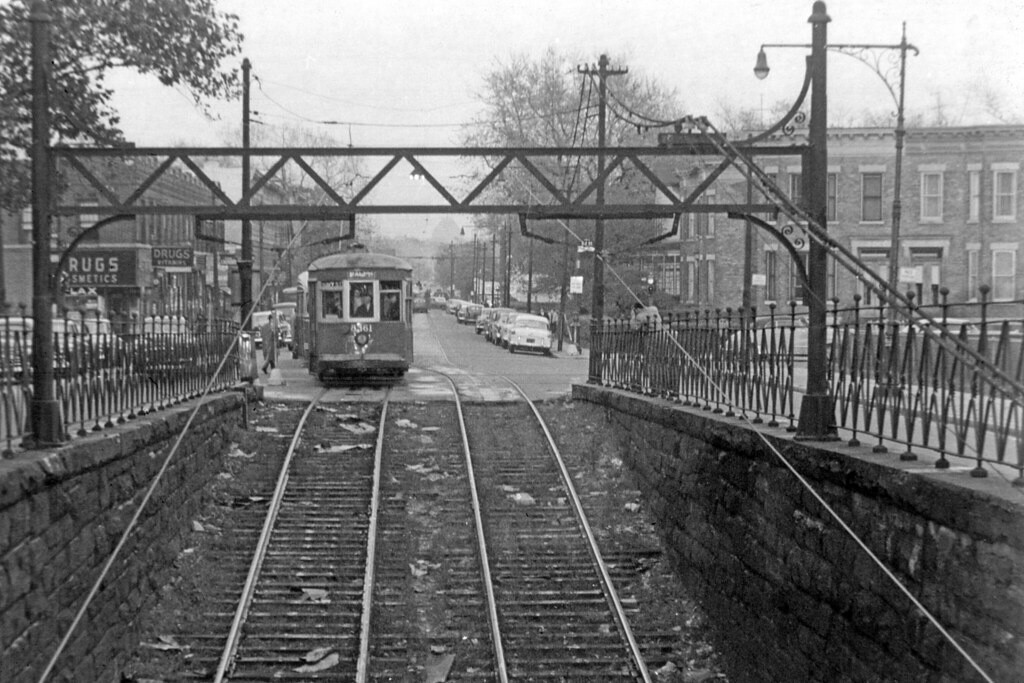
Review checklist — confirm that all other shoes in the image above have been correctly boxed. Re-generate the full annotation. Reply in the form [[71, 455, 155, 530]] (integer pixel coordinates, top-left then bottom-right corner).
[[261, 367, 268, 374]]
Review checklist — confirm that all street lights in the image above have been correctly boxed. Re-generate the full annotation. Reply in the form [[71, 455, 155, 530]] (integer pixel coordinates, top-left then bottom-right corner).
[[460, 224, 513, 308], [754, 0, 920, 442]]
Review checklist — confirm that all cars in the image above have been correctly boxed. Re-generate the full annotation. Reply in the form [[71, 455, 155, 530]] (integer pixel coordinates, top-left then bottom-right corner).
[[429, 297, 552, 355], [0, 288, 298, 387]]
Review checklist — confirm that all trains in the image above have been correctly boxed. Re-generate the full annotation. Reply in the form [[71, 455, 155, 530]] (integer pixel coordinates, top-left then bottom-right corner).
[[295, 249, 415, 390]]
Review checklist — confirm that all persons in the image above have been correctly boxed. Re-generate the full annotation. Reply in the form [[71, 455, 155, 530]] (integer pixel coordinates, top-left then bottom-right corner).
[[261, 314, 274, 374], [630, 301, 663, 395], [539, 307, 557, 333], [387, 294, 399, 321], [356, 295, 373, 318]]
